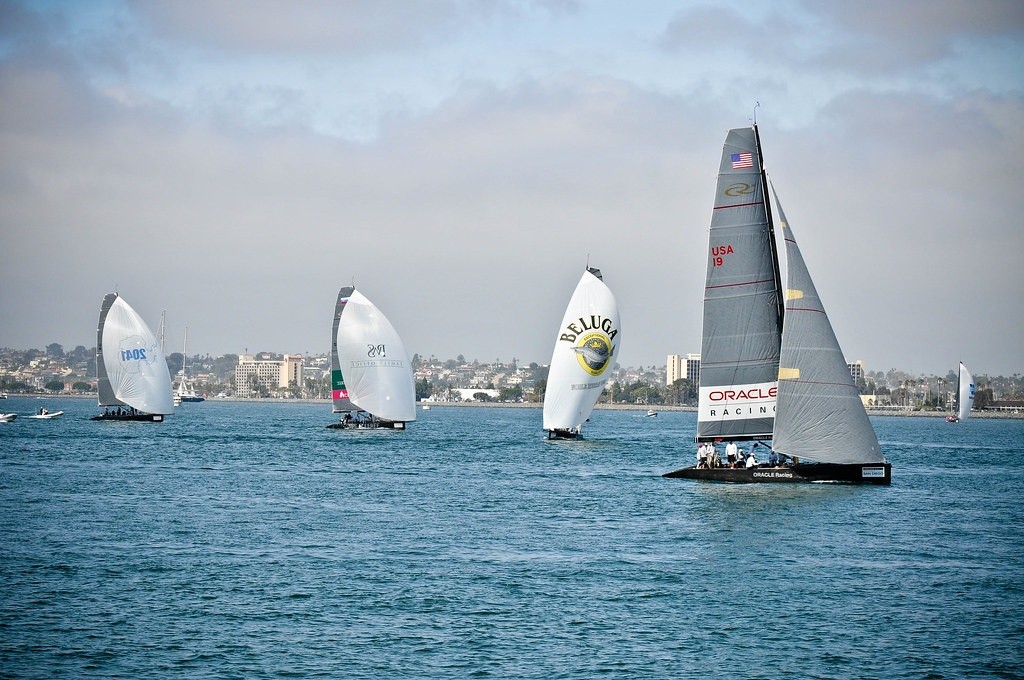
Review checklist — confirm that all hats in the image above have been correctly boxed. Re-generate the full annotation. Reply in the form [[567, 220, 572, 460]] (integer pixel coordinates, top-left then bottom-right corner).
[[750, 453, 755, 456]]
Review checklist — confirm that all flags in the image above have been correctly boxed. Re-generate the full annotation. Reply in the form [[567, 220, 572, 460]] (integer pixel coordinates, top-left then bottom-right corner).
[[730, 152, 752, 169], [958, 363, 974, 421]]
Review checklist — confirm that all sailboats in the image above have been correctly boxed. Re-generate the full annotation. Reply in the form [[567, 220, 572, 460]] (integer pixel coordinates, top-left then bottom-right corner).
[[325, 275, 417, 432], [175, 323, 205, 402], [945, 361, 975, 423], [156, 307, 181, 407], [662, 99, 893, 488], [92, 286, 176, 423], [542, 251, 621, 443]]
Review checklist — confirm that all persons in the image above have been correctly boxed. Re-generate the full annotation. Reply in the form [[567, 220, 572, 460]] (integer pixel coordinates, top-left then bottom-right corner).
[[736, 443, 788, 469], [647, 409, 651, 416], [99, 406, 137, 417], [564, 428, 582, 435], [695, 441, 720, 469], [725, 440, 737, 468], [38, 406, 45, 415], [339, 415, 370, 425]]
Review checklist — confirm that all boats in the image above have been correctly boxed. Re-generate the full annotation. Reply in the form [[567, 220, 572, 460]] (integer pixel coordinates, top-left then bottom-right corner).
[[0, 413, 18, 423], [644, 410, 657, 418], [20, 411, 64, 419], [0, 393, 7, 399]]
